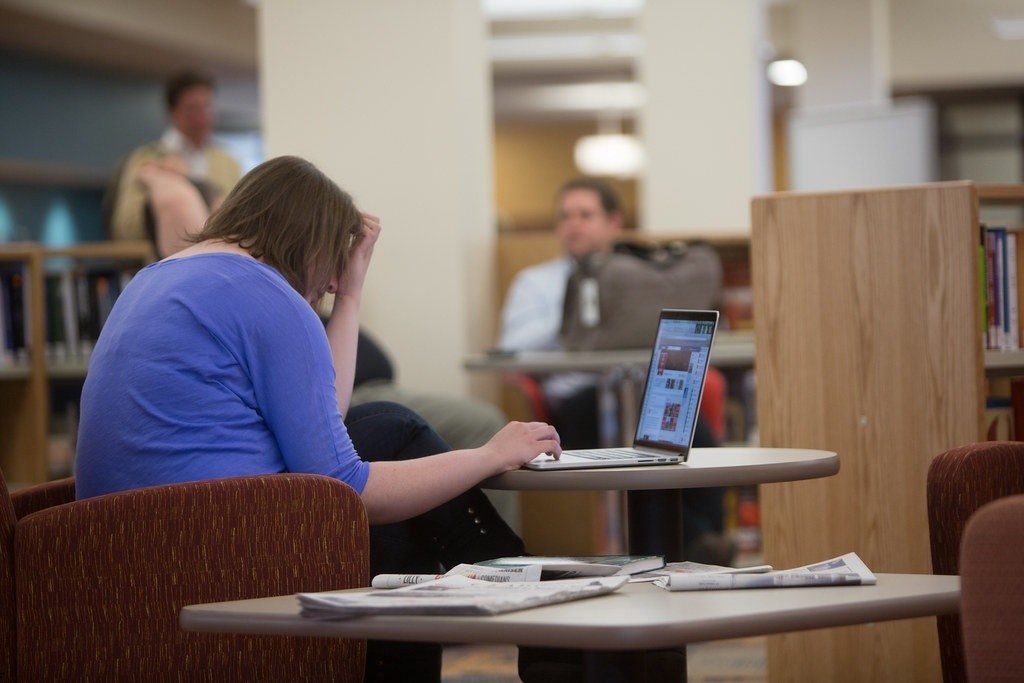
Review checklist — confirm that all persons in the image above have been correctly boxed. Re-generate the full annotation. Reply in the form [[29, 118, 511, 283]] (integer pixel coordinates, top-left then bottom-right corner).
[[107, 69, 738, 566], [75, 156, 562, 683]]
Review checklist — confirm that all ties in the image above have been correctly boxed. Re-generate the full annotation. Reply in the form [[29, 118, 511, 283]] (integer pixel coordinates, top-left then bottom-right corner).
[[559, 269, 584, 337]]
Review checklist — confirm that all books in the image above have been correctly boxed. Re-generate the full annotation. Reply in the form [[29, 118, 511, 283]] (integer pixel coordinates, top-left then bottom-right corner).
[[980, 218, 1024, 444], [0, 254, 145, 486]]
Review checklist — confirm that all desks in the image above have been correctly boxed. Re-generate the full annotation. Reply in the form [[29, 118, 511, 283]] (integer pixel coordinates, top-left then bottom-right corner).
[[460, 328, 758, 560], [180, 561, 963, 683], [477, 447, 840, 556]]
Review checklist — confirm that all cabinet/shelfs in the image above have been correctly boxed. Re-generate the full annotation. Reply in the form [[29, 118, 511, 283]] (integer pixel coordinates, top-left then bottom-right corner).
[[749, 181, 1024, 683], [0, 238, 154, 493]]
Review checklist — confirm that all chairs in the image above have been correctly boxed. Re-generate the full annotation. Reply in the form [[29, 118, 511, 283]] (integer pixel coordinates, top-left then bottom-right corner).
[[926, 440, 1024, 683], [0, 465, 372, 683]]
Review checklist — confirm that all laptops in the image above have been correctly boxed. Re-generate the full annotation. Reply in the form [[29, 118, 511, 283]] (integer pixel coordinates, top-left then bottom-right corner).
[[523, 308, 721, 470]]
